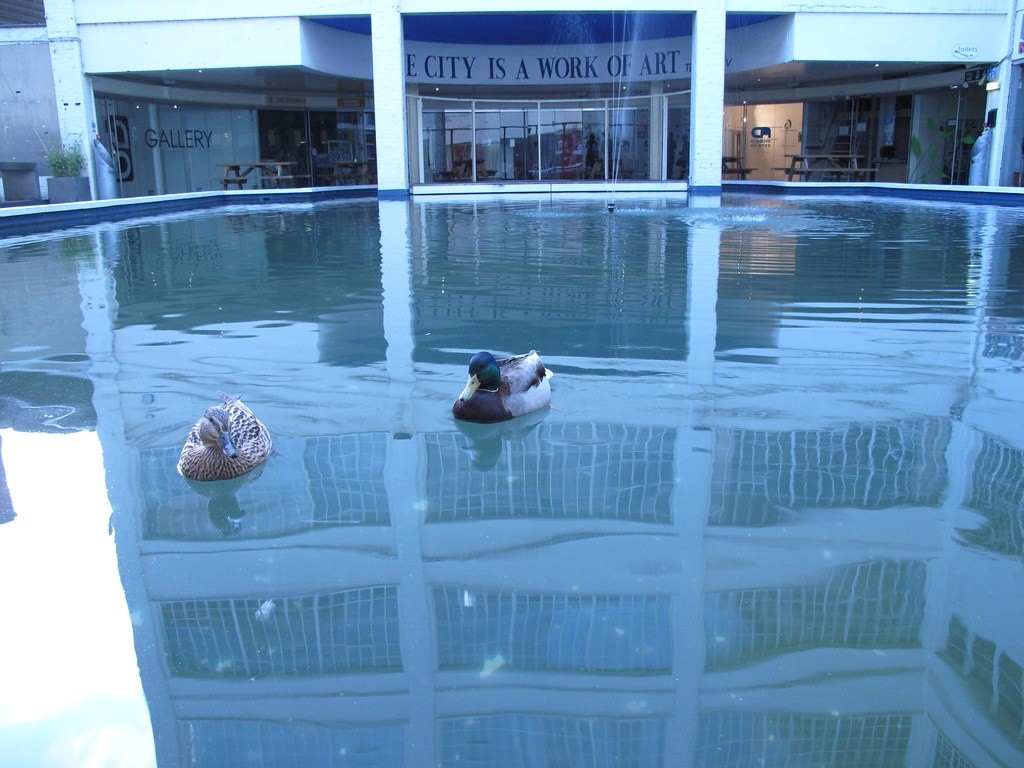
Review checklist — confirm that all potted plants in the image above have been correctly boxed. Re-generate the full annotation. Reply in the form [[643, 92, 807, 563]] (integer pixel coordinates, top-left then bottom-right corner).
[[44, 134, 92, 204]]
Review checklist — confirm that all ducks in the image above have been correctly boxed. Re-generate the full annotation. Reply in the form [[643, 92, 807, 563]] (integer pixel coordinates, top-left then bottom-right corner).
[[176, 391, 273, 481]]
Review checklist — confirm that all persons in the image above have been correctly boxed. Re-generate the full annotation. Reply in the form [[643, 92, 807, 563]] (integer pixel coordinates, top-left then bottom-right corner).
[[583, 133, 614, 180], [667, 132, 689, 180]]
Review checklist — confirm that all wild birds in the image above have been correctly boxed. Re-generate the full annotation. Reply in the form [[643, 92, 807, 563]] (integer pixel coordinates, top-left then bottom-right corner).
[[453, 350, 553, 420]]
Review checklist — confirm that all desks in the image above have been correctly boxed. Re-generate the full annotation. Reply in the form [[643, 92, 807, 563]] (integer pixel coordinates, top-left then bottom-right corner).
[[451, 160, 488, 182], [722, 156, 747, 180], [333, 162, 376, 184], [216, 161, 298, 188], [784, 154, 864, 181]]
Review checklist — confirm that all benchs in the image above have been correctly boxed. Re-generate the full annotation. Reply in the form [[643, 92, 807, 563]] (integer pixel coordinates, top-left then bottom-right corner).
[[772, 167, 878, 172], [440, 171, 457, 178], [722, 168, 758, 174], [214, 175, 309, 182], [477, 169, 498, 176], [318, 172, 376, 179]]
